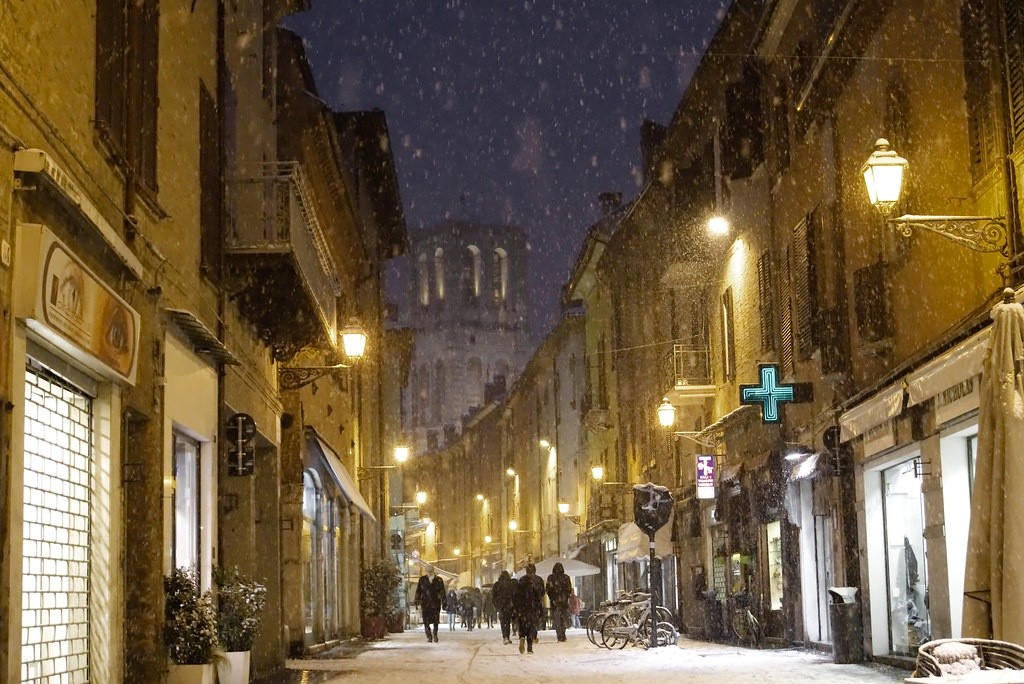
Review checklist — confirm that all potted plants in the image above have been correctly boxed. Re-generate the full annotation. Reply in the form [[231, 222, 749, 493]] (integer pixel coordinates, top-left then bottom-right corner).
[[388, 567, 411, 633], [361, 560, 390, 639], [216, 568, 266, 684], [159, 571, 215, 684]]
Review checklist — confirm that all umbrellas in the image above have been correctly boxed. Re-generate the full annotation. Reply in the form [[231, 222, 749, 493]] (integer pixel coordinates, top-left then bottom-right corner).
[[511, 557, 601, 579], [960, 286, 1023, 650]]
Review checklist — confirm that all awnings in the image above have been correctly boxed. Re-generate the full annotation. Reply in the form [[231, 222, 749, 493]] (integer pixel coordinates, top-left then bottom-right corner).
[[616, 505, 673, 564], [304, 422, 377, 524]]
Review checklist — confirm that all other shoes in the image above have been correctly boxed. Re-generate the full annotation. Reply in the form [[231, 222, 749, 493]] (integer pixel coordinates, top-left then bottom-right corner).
[[503, 637, 512, 645], [434, 636, 438, 642], [527, 651, 533, 654], [533, 639, 538, 643], [428, 637, 432, 642], [519, 637, 525, 654], [558, 637, 567, 642]]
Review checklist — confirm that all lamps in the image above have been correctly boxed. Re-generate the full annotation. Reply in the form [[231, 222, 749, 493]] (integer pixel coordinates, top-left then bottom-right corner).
[[655, 397, 676, 429], [858, 138, 1007, 252], [706, 129, 730, 236], [358, 441, 409, 480], [340, 273, 368, 363]]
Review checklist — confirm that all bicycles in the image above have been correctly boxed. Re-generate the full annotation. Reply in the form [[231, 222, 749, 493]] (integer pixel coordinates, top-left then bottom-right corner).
[[587, 588, 677, 651], [730, 588, 761, 648]]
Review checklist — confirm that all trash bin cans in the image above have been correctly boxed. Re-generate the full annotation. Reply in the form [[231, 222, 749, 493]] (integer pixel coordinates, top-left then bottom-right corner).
[[827, 586, 864, 664]]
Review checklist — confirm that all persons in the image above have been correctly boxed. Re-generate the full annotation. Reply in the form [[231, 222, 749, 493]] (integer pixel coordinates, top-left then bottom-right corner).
[[413, 564, 447, 643], [443, 561, 583, 653]]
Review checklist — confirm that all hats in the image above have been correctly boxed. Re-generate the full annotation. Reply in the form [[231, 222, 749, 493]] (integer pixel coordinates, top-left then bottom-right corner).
[[425, 565, 434, 572]]
